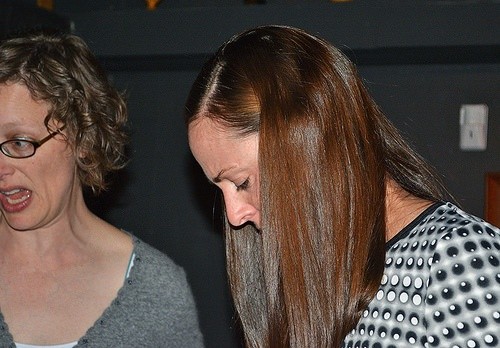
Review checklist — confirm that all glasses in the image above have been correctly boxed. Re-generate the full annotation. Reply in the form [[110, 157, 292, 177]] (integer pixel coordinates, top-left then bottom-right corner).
[[0, 123, 70, 159]]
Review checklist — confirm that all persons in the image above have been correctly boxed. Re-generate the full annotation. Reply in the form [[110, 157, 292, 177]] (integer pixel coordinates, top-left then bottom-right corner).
[[0, 34, 205, 348], [184, 23, 500, 347]]
